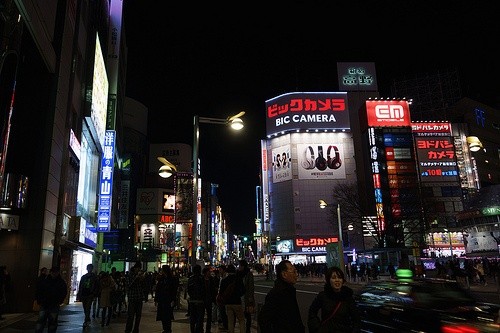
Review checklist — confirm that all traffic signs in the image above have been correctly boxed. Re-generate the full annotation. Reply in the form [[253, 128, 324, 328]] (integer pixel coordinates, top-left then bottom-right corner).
[[238, 235, 249, 241]]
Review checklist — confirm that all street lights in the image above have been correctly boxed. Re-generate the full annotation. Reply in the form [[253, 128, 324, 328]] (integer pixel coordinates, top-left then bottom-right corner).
[[159, 111, 246, 268], [255, 220, 280, 279], [320, 201, 353, 278], [470, 143, 481, 153]]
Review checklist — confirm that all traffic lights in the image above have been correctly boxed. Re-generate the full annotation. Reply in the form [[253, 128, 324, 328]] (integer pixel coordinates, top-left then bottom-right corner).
[[197, 246, 202, 250], [176, 237, 180, 242]]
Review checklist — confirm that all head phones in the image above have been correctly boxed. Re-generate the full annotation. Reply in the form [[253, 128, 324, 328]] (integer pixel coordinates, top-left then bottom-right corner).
[[315, 145, 327, 171], [301, 146, 315, 169], [326, 146, 342, 169]]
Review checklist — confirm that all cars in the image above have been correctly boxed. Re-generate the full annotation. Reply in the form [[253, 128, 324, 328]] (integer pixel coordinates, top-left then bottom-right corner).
[[355, 281, 500, 333]]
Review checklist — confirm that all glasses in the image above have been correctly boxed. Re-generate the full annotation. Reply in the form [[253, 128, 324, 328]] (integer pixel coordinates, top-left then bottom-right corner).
[[285, 270, 297, 273]]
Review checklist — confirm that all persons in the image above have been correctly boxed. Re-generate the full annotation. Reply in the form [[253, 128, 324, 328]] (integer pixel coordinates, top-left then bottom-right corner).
[[434, 258, 500, 287], [266, 260, 306, 333], [253, 261, 395, 280], [35, 260, 256, 333], [0, 266, 11, 320], [309, 266, 360, 333]]
